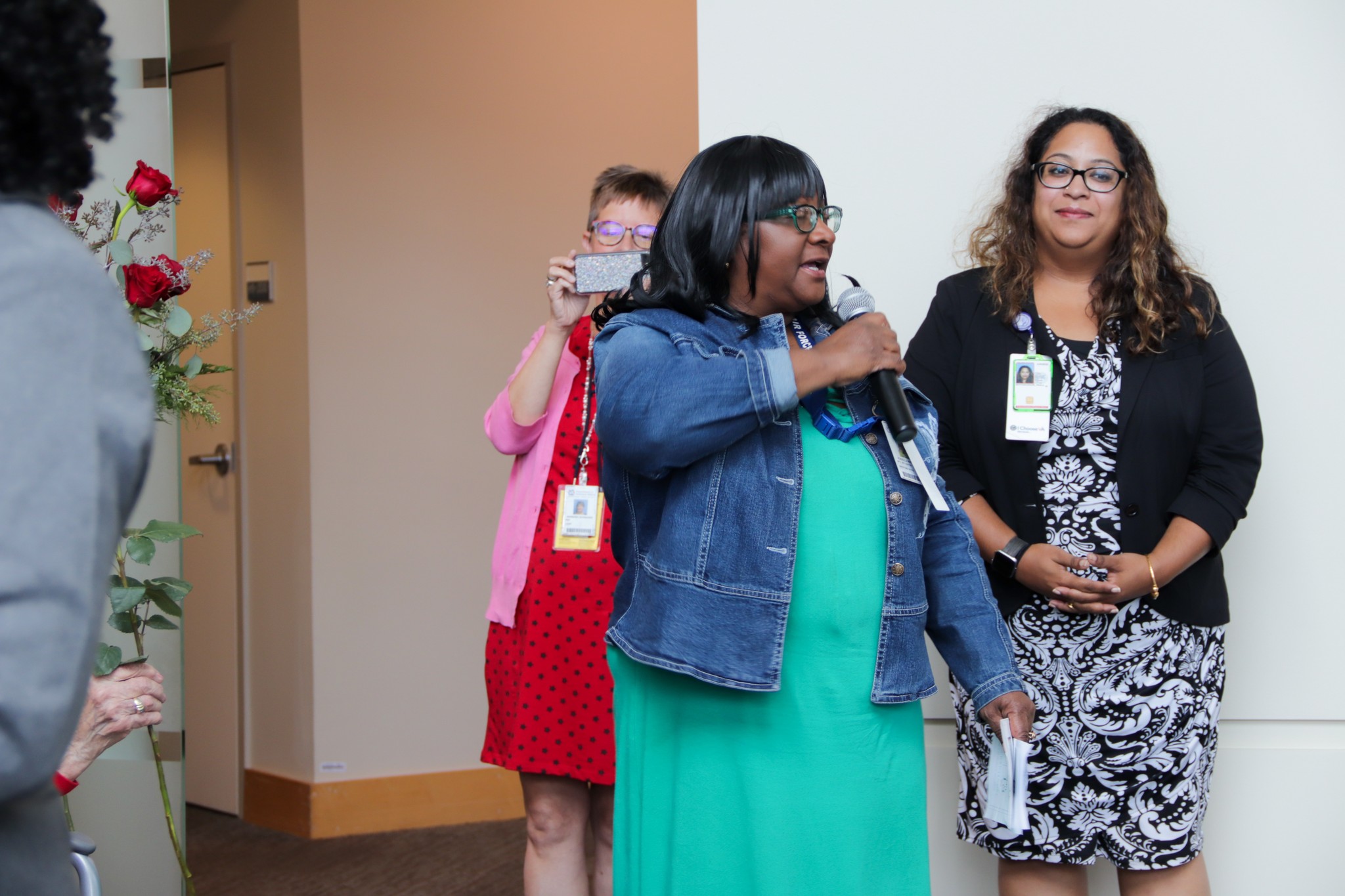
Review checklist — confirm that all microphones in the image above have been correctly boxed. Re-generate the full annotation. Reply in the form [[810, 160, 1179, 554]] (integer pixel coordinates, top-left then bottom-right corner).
[[836, 287, 918, 443]]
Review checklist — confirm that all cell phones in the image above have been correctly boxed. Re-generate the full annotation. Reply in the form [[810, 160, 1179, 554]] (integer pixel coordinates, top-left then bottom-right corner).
[[572, 251, 652, 295]]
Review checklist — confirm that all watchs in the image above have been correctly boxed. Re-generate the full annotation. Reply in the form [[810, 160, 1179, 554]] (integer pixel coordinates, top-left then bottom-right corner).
[[989, 536, 1031, 583]]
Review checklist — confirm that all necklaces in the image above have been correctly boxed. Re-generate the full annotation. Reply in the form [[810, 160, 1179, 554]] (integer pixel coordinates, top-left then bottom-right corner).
[[583, 317, 597, 466]]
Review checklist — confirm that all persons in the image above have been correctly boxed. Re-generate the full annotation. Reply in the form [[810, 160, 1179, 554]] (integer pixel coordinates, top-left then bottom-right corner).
[[590, 133, 1037, 896], [51, 662, 167, 795], [896, 108, 1264, 896], [482, 163, 674, 896], [0, 0, 158, 895]]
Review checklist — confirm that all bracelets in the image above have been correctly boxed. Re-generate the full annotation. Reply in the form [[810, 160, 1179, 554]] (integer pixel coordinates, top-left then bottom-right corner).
[[1146, 555, 1160, 602]]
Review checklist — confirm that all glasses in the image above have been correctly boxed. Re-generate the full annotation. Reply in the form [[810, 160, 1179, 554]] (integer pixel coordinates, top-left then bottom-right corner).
[[742, 204, 843, 233], [590, 221, 658, 249], [1030, 162, 1129, 193]]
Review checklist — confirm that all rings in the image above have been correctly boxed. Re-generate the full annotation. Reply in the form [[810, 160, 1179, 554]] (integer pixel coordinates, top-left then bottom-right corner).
[[133, 697, 145, 714], [545, 274, 558, 287]]
